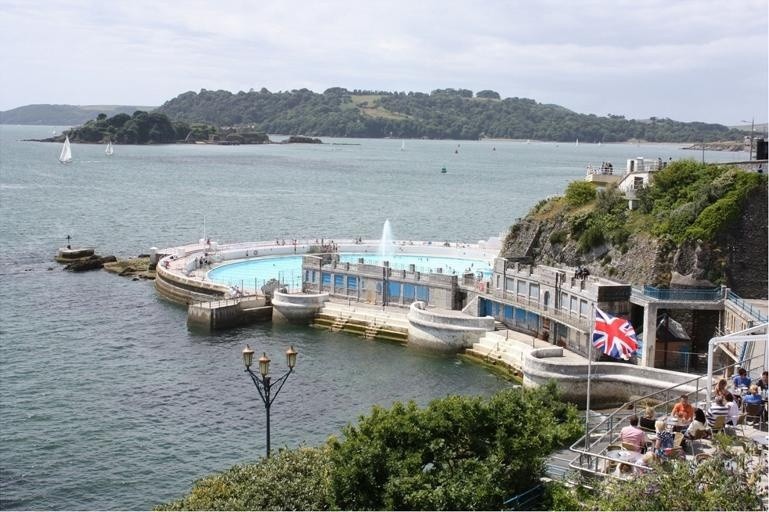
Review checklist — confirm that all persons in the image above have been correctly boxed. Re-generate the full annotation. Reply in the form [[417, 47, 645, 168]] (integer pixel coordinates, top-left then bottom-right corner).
[[581, 268, 590, 280], [618, 369, 768, 456], [575, 266, 582, 279]]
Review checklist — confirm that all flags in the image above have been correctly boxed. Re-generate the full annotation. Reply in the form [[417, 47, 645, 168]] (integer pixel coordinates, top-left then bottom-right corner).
[[592, 305, 638, 361]]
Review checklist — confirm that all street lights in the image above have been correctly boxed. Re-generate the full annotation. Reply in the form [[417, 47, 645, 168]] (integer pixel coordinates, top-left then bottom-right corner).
[[243, 344, 298, 459], [742, 119, 754, 160]]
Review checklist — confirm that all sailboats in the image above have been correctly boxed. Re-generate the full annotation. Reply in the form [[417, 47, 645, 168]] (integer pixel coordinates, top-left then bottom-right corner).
[[106, 140, 113, 155], [59, 135, 72, 164]]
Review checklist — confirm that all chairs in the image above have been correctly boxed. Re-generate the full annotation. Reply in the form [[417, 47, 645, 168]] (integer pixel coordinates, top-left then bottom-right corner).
[[606, 385, 768, 479]]
